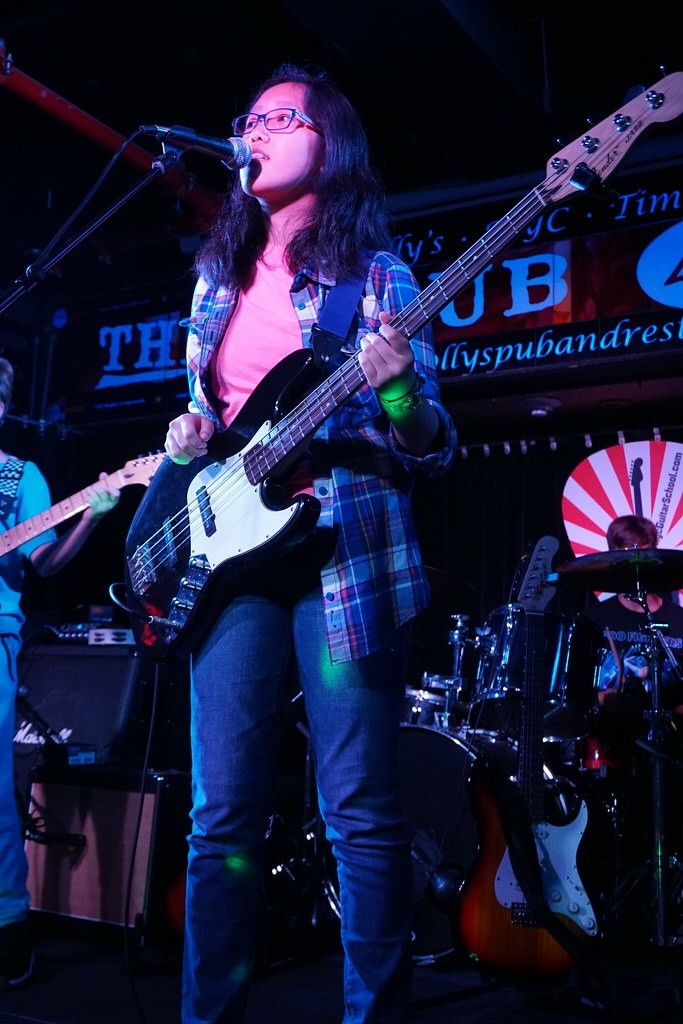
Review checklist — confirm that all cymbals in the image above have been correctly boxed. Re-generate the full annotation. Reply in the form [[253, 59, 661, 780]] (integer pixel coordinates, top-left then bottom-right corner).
[[554, 548, 683, 594]]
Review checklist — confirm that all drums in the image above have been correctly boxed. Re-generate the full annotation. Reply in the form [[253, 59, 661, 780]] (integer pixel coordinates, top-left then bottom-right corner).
[[465, 603, 608, 730], [309, 724, 480, 972], [399, 687, 468, 737]]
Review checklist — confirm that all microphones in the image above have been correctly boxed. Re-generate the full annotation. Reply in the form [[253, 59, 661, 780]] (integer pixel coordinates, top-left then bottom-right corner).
[[427, 870, 466, 900], [140, 126, 252, 171]]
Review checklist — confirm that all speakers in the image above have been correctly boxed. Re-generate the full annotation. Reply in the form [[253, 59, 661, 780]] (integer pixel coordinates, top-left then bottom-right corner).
[[12, 645, 191, 787], [21, 773, 193, 932]]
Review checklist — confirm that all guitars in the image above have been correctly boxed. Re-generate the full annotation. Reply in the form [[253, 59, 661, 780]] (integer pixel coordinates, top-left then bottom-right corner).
[[456, 536, 601, 982], [124, 69, 682, 665], [0, 448, 170, 558]]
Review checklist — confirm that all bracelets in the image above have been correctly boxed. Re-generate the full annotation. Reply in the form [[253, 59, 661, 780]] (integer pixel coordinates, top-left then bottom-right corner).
[[380, 371, 426, 413]]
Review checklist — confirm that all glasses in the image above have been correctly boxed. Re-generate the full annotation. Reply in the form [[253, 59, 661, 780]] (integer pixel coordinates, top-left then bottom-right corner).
[[231, 108, 322, 136]]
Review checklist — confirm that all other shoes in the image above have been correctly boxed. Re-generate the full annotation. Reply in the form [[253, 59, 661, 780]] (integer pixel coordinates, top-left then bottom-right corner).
[[0, 920, 37, 987]]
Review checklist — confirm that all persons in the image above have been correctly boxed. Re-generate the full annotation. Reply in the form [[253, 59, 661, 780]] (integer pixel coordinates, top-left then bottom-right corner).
[[0, 358, 120, 984], [164, 65, 460, 1024], [593, 514, 683, 714]]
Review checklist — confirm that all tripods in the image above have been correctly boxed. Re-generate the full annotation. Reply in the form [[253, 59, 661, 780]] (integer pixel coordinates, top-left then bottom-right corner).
[[602, 563, 683, 949]]
